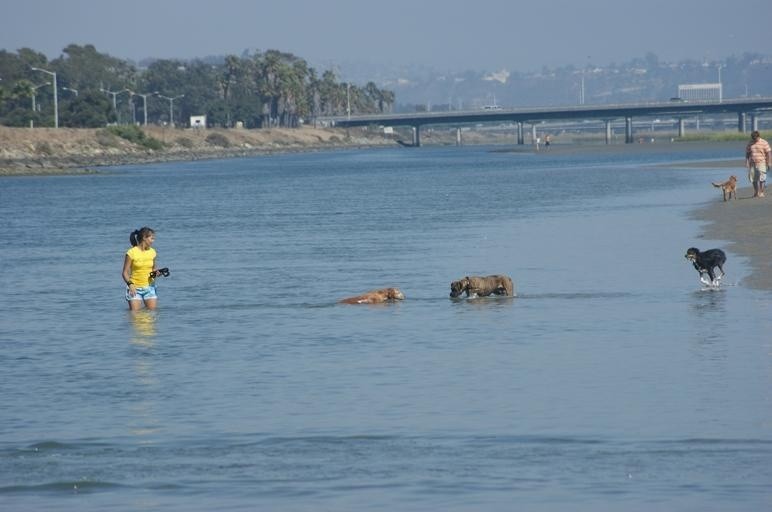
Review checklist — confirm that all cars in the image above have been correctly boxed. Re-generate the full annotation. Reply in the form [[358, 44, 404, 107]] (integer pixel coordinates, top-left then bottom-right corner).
[[669, 97, 690, 103], [480, 104, 504, 112]]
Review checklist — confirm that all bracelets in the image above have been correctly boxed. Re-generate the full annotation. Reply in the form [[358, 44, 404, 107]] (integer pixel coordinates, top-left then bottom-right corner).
[[127, 281, 134, 286]]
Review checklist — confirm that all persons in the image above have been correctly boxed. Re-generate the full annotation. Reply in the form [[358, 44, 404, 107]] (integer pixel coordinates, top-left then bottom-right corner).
[[121, 226, 160, 311], [126, 312, 158, 407], [744, 131, 772, 200], [535, 137, 541, 151], [544, 135, 551, 150]]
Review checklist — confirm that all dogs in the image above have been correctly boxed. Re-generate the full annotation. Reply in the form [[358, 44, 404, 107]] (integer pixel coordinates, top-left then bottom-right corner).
[[450, 274, 514, 300], [338, 287, 406, 304], [684, 246, 727, 287], [711, 174, 739, 201]]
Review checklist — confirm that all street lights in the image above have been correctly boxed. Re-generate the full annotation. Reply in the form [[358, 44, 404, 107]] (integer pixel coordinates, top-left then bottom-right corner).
[[718, 64, 727, 103], [25, 63, 189, 129]]
